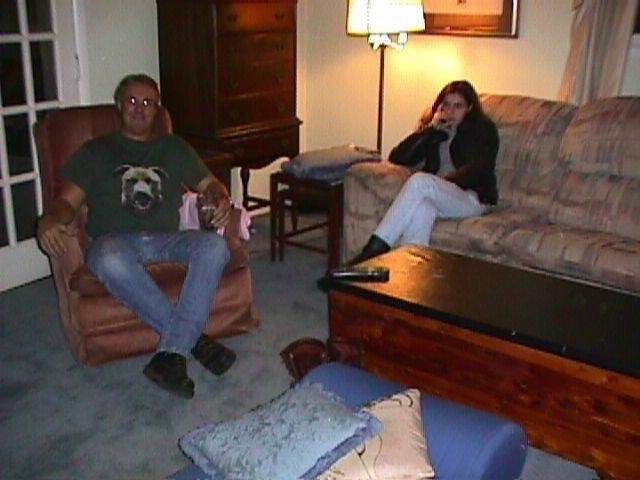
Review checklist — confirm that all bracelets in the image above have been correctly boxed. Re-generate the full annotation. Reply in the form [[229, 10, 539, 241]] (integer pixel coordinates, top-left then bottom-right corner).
[[214, 191, 233, 205]]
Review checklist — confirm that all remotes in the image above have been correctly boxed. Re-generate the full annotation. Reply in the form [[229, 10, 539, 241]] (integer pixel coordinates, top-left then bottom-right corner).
[[330, 265, 391, 282]]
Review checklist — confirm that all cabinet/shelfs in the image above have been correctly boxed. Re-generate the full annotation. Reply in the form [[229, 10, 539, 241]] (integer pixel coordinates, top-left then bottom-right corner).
[[156, 0, 303, 231]]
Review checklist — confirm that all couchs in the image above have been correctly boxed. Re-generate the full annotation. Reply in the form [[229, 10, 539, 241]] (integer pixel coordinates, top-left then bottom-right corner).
[[344, 94, 640, 297]]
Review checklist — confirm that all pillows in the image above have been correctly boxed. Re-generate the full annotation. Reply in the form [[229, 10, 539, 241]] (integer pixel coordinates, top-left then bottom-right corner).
[[177, 380, 437, 480]]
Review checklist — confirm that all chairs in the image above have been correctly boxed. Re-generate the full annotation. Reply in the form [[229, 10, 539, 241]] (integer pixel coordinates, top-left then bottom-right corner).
[[165, 361, 528, 480], [32, 102, 258, 367]]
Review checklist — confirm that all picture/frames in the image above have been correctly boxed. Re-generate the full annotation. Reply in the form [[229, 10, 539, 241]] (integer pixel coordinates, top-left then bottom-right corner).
[[418, 0, 520, 38]]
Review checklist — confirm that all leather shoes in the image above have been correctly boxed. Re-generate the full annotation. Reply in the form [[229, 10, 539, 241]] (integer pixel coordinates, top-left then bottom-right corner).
[[191, 333, 235, 375], [143, 351, 194, 398]]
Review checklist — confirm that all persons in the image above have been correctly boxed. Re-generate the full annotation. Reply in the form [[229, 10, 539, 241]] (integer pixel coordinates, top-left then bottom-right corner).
[[342, 78, 500, 274], [32, 72, 238, 401]]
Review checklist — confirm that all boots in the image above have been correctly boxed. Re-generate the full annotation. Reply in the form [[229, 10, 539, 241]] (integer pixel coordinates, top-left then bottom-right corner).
[[317, 236, 390, 290]]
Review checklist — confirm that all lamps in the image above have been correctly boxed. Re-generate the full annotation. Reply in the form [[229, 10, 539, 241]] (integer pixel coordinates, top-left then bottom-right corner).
[[346, 0, 425, 157]]
